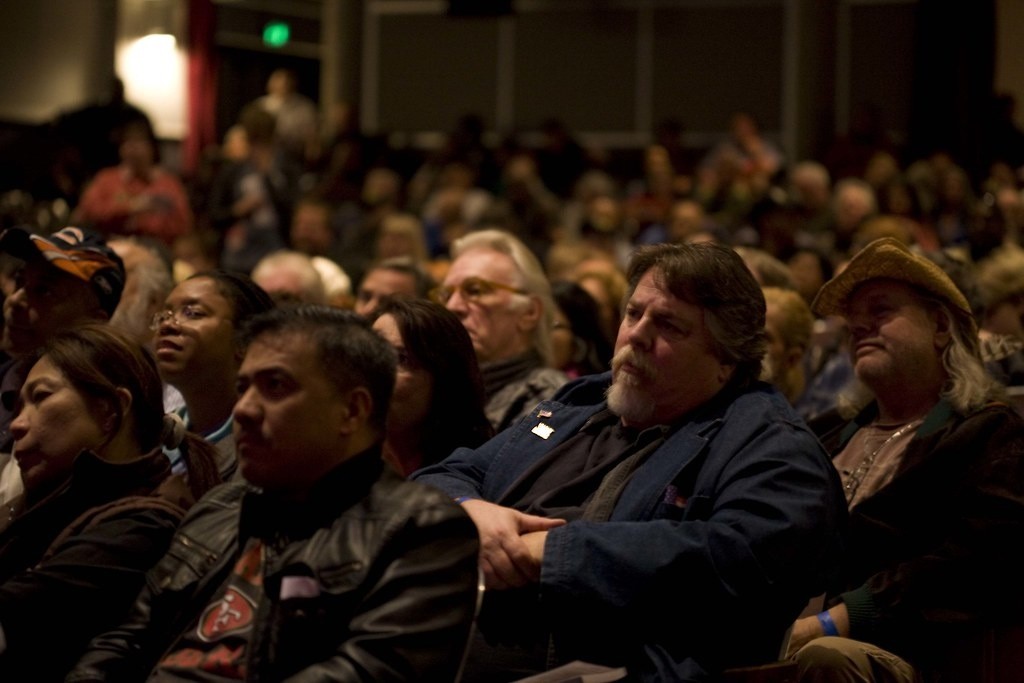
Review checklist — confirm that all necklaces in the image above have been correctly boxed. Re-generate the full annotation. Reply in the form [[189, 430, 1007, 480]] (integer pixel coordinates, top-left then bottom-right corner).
[[843, 415, 921, 490]]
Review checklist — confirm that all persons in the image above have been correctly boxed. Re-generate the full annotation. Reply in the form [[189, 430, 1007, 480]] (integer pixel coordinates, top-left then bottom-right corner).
[[0, 66, 1024, 683]]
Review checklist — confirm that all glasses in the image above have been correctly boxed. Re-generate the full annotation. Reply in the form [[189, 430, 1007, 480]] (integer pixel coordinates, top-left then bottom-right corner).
[[148, 306, 233, 331], [439, 276, 527, 304]]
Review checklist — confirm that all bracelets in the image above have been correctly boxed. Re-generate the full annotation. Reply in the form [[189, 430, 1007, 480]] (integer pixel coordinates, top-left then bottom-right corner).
[[817, 609, 840, 635], [455, 496, 473, 506]]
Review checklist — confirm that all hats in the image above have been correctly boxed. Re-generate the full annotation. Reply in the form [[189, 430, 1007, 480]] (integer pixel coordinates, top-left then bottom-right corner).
[[810, 238, 979, 347], [0, 226, 126, 319]]
